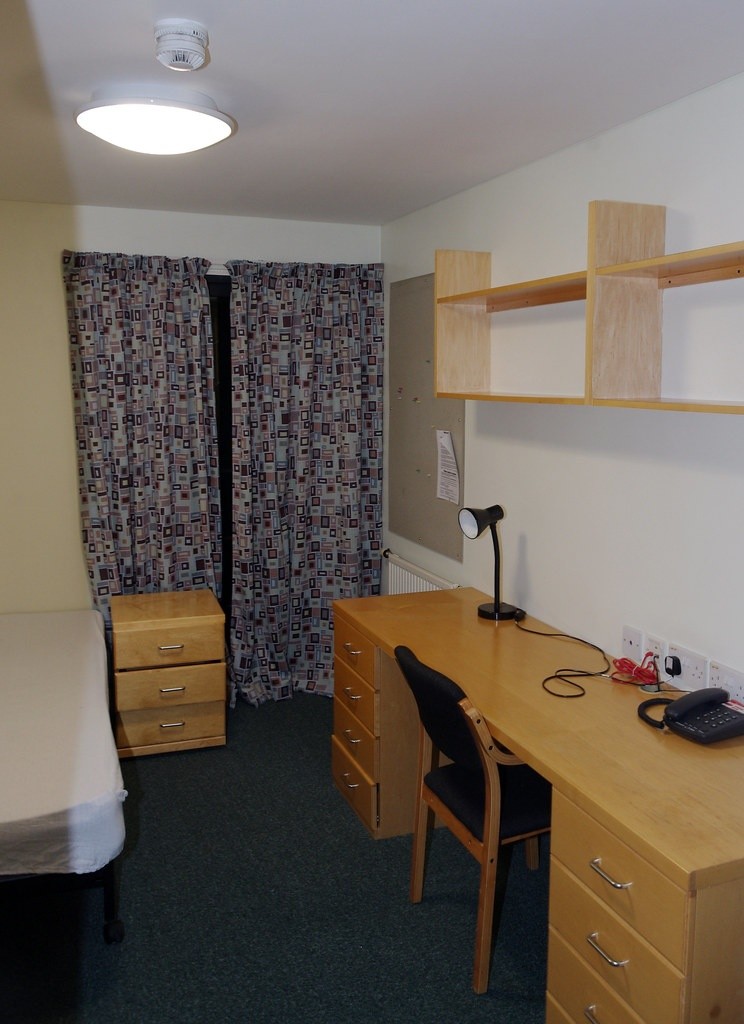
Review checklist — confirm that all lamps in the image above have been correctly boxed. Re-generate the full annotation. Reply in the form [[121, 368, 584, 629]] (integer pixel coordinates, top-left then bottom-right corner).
[[459, 505, 518, 621], [74, 81, 239, 157]]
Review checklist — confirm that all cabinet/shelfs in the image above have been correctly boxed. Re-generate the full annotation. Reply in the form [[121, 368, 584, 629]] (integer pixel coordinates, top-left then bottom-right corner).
[[433, 199, 744, 417], [328, 584, 744, 1024]]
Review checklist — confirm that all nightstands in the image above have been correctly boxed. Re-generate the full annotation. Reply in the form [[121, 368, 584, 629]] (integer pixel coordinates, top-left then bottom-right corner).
[[107, 587, 232, 759]]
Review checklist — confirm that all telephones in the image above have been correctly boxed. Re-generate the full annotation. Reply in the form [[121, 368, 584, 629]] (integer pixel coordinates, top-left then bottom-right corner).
[[664, 687, 744, 745]]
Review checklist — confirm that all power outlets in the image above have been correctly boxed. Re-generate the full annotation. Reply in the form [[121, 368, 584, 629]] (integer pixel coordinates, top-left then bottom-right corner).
[[707, 660, 744, 706], [665, 643, 708, 691], [621, 624, 644, 662], [640, 631, 668, 676]]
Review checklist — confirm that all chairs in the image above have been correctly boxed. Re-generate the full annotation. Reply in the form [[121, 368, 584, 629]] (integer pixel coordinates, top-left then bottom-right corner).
[[392, 644, 553, 998]]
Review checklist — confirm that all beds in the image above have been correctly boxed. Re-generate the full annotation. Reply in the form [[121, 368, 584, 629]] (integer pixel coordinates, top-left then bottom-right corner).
[[0, 609, 130, 952]]
